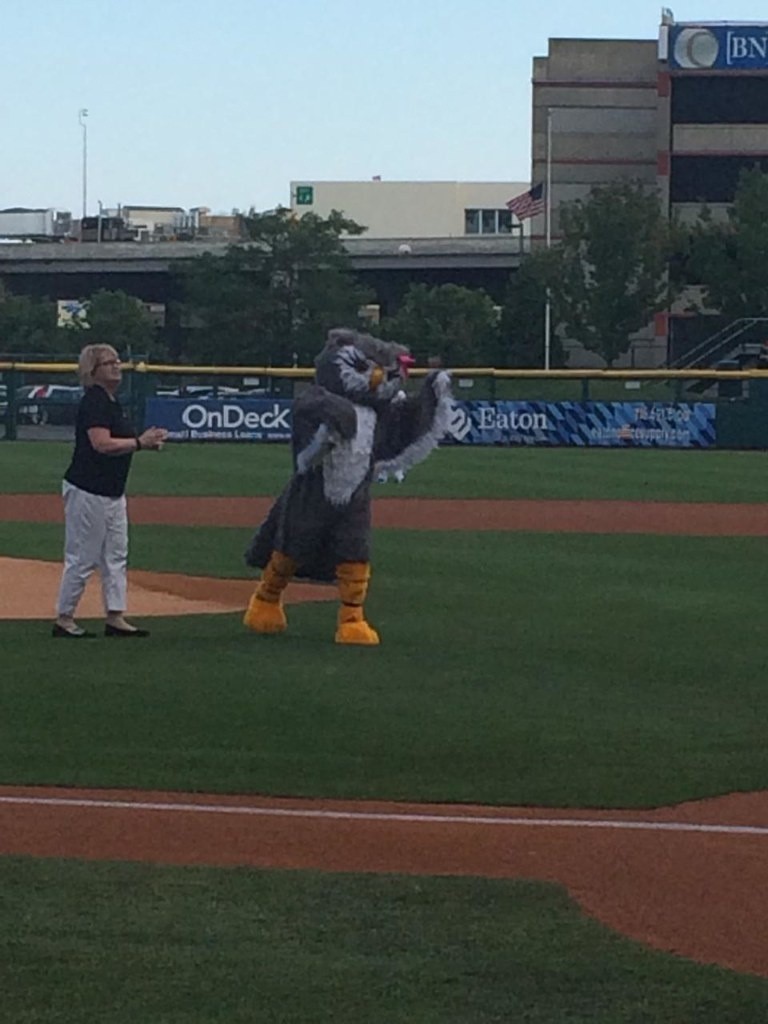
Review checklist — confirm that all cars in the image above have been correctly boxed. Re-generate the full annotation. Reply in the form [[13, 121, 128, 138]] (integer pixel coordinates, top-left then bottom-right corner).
[[0, 383, 84, 425]]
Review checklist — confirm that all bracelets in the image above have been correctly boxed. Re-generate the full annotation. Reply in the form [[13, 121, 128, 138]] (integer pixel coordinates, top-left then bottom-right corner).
[[136, 437, 141, 451]]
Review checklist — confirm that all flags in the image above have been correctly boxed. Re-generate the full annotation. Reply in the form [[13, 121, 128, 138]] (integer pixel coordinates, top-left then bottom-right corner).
[[506, 183, 544, 222]]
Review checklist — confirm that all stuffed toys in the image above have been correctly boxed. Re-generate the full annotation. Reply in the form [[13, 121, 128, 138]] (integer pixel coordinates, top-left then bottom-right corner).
[[243, 329, 451, 645]]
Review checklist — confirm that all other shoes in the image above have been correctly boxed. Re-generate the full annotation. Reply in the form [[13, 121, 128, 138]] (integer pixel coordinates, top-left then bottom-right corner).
[[52, 623, 97, 638], [104, 623, 150, 638]]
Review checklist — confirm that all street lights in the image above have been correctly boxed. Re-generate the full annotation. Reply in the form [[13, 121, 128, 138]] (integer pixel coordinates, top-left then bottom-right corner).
[[77, 110, 90, 249]]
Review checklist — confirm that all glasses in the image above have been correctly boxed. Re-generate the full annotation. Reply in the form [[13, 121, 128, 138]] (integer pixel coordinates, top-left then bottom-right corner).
[[97, 357, 121, 366]]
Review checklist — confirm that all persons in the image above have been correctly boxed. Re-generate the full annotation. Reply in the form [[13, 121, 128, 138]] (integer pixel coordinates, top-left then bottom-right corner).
[[51, 346, 168, 639]]
